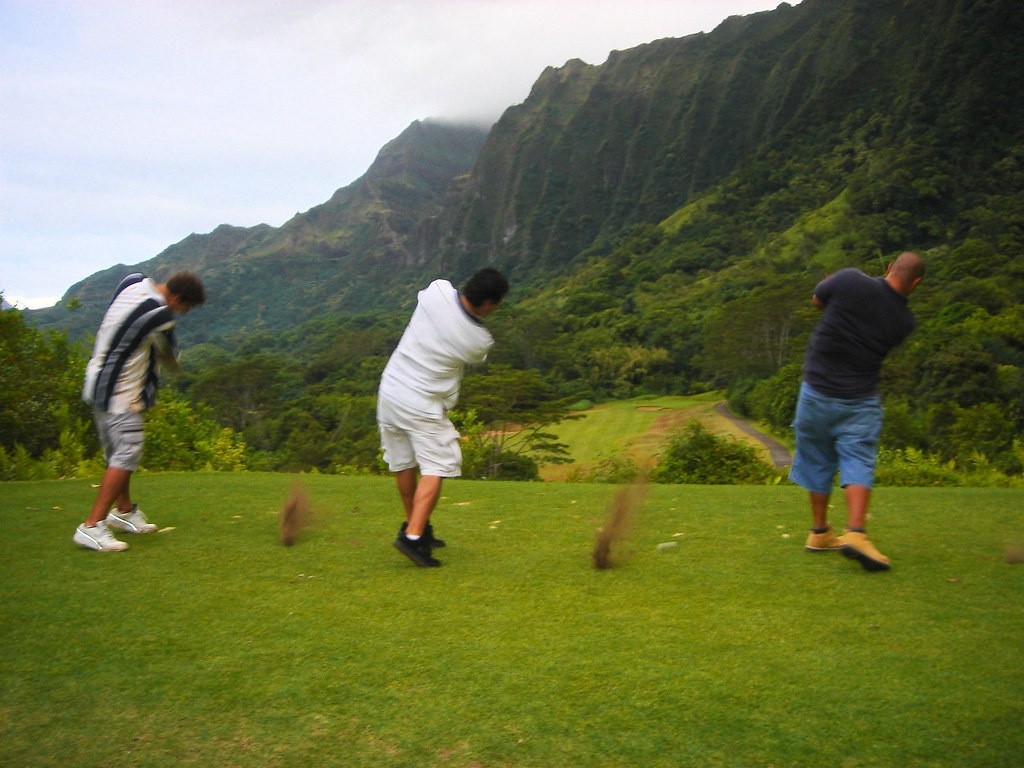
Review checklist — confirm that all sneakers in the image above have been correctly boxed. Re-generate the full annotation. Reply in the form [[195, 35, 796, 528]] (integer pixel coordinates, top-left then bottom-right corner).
[[73, 520, 128, 552], [398, 522, 446, 547], [840, 532, 891, 571], [393, 531, 441, 567], [106, 504, 158, 534], [804, 525, 845, 551]]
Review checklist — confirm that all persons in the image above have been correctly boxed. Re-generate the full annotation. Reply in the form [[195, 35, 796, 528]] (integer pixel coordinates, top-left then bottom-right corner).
[[788, 252, 927, 572], [72, 271, 206, 551], [377, 269, 510, 568]]
